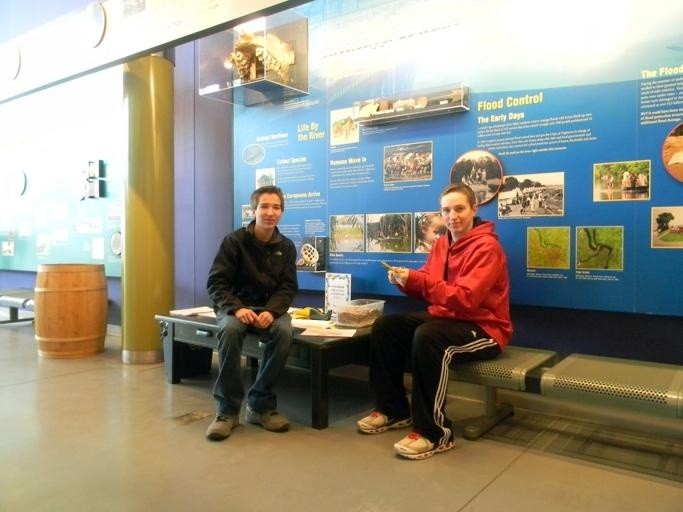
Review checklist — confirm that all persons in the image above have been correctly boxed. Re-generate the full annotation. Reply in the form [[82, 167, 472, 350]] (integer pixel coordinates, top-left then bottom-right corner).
[[414, 213, 443, 252], [606, 171, 647, 186], [461, 166, 488, 186], [207, 185, 298, 439], [358, 183, 513, 460], [499, 191, 546, 215]]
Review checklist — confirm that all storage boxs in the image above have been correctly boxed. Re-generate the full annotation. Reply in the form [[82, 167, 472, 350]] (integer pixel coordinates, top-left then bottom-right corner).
[[333, 298, 386, 329]]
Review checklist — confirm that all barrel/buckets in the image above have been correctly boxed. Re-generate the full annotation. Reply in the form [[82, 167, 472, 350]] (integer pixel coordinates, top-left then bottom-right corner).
[[34, 262, 107, 358]]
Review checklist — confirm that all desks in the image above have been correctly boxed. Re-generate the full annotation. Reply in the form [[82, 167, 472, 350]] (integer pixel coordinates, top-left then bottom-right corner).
[[153, 306, 379, 430]]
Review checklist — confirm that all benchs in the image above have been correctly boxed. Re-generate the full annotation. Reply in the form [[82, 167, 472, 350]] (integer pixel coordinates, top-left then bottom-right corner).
[[1, 287, 38, 325], [448, 344, 683, 441]]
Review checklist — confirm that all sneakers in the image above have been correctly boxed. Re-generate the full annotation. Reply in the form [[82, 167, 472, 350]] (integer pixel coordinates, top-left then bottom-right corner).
[[206, 413, 239, 437], [357, 410, 413, 434], [245, 403, 290, 431], [393, 431, 456, 460]]
